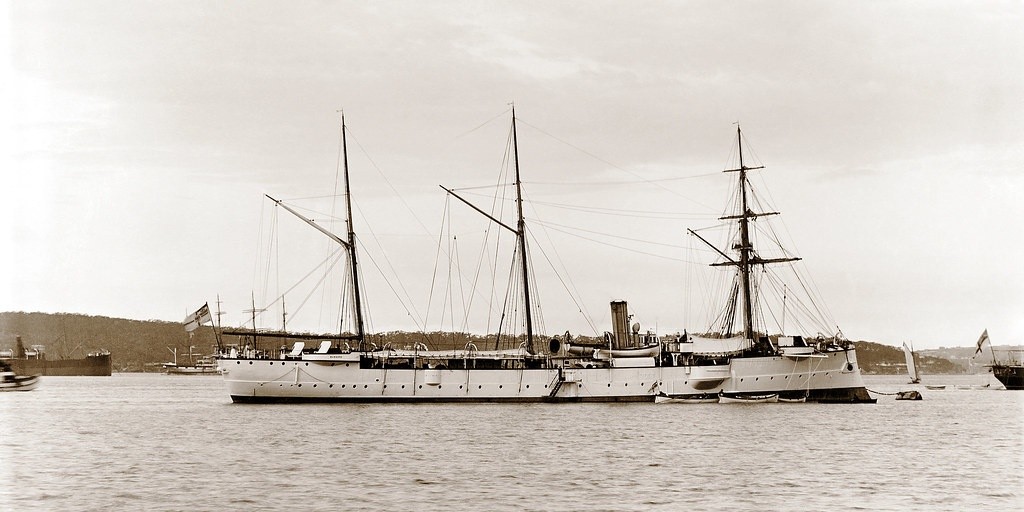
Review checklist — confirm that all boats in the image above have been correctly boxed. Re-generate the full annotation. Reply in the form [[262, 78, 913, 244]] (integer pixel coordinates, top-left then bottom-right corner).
[[162, 344, 220, 374], [214, 101, 880, 403], [0, 371, 40, 391], [0, 334, 112, 377], [983, 341, 1024, 389], [925, 385, 946, 391]]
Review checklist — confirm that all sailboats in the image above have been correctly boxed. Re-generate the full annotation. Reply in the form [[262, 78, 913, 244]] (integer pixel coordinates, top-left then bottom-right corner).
[[903, 341, 922, 384]]
